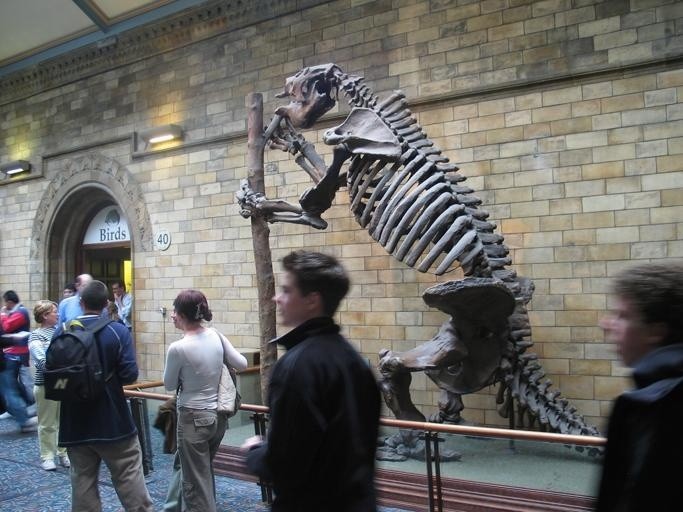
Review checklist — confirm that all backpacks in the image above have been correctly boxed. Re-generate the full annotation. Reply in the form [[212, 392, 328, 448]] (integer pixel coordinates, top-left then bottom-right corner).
[[42, 317, 115, 402]]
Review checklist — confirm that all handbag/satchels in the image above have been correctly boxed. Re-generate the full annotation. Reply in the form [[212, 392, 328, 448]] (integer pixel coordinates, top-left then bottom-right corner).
[[218, 361, 241, 417]]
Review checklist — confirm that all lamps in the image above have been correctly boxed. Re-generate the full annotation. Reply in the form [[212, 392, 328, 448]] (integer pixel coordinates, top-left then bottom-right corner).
[[139, 123, 183, 145], [0, 159, 30, 175]]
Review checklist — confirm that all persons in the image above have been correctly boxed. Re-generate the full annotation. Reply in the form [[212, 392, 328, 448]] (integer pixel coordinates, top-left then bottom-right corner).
[[43, 280, 154, 512], [29, 300, 70, 470], [59, 272, 135, 331], [162, 298, 182, 512], [243, 248, 377, 512], [0, 291, 32, 434], [153, 288, 247, 512], [591, 263, 683, 511]]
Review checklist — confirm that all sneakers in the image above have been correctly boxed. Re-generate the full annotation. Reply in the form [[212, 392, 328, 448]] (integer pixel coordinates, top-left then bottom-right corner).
[[39, 459, 57, 471], [61, 457, 70, 468]]
